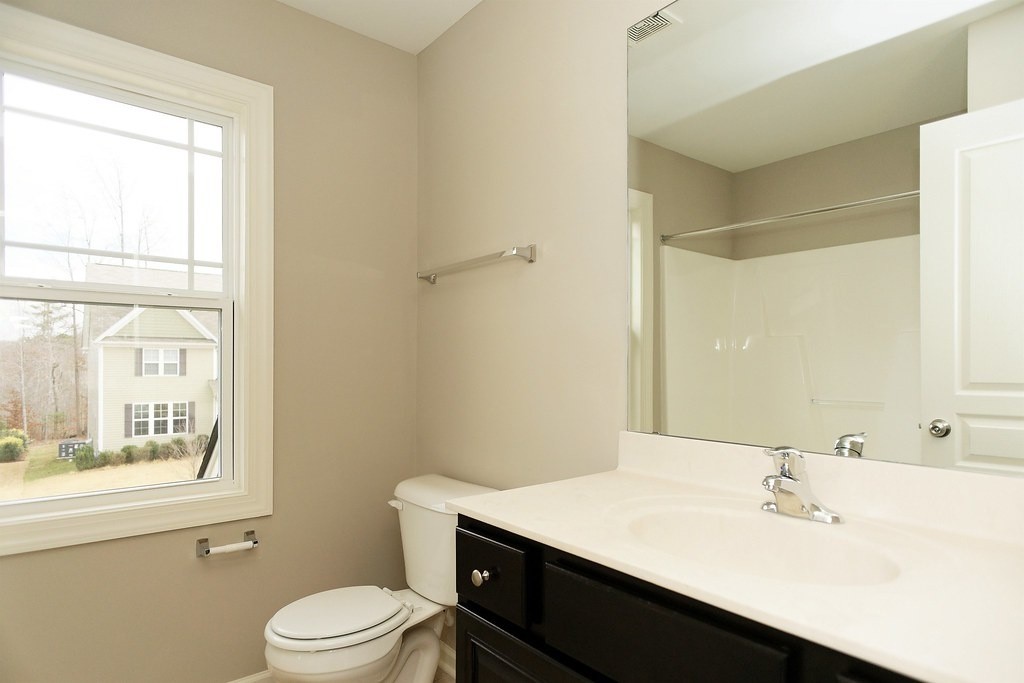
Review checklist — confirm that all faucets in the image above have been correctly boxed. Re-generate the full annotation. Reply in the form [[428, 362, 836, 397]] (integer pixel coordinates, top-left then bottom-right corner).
[[834, 431, 868, 456], [762, 449, 837, 523]]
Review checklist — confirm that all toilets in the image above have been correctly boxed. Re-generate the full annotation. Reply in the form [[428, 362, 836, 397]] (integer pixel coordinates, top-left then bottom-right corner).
[[263, 472, 504, 683]]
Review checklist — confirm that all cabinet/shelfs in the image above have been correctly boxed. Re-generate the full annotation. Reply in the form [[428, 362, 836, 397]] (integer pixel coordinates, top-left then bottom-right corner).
[[455, 515, 1023, 683]]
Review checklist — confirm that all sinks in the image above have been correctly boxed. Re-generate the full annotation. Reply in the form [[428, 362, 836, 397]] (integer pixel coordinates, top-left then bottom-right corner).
[[589, 502, 897, 613]]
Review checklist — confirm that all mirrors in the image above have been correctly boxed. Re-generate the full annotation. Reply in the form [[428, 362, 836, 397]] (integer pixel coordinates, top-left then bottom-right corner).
[[626, 0, 1024, 478]]
